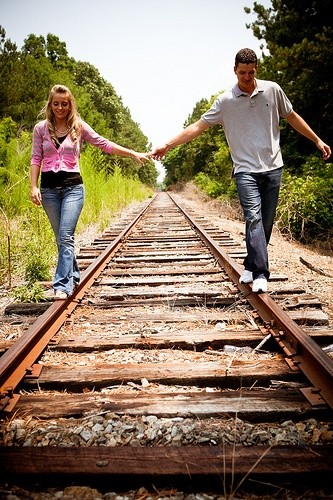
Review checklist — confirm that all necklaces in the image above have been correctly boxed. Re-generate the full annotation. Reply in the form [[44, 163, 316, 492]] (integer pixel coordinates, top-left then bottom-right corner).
[[54, 126, 68, 134]]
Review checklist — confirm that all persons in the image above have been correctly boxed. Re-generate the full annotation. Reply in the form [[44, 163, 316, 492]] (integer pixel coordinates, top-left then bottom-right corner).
[[30, 84, 154, 300], [149, 48, 331, 293]]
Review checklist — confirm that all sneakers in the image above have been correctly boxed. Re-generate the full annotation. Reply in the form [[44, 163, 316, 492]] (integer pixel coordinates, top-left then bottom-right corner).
[[251, 278, 268, 292], [239, 270, 253, 283]]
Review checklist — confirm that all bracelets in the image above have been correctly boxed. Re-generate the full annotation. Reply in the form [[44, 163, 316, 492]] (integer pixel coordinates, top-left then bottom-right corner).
[[128, 149, 134, 160]]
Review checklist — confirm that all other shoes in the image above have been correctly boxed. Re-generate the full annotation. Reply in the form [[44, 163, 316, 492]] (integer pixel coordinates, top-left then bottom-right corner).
[[52, 291, 67, 299]]
